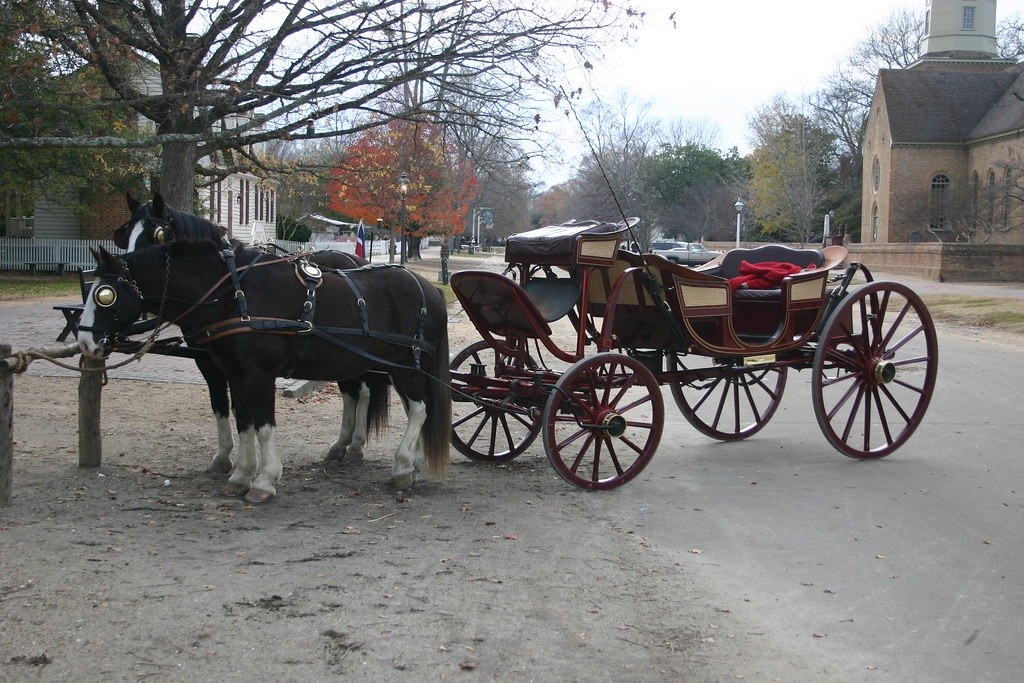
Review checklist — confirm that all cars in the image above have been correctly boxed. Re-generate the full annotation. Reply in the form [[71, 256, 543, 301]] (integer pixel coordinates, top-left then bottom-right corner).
[[651, 241, 725, 267]]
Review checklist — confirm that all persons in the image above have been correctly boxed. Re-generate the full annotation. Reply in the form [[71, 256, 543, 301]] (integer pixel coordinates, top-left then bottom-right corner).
[[630, 238, 640, 253], [621, 244, 626, 249]]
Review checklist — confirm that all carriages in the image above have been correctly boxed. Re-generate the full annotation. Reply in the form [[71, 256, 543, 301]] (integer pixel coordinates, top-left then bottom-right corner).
[[74, 193, 942, 502]]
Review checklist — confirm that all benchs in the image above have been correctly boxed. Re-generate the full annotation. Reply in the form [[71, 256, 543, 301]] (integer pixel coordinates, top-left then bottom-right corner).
[[24, 262, 70, 276], [698, 246, 849, 302], [576, 249, 732, 318]]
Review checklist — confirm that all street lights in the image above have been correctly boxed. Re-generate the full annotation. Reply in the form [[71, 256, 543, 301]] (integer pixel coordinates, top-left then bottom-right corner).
[[734, 197, 744, 248], [829, 209, 835, 235], [399, 172, 411, 266]]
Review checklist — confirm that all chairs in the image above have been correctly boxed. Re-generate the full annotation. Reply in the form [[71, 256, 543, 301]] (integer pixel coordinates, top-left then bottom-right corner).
[[450, 218, 640, 363]]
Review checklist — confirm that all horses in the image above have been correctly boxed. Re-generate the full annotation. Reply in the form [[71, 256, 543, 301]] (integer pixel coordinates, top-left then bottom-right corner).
[[76, 190, 453, 503]]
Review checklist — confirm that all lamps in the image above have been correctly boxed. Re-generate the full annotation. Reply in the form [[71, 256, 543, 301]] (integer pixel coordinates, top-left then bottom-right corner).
[[237, 196, 242, 204]]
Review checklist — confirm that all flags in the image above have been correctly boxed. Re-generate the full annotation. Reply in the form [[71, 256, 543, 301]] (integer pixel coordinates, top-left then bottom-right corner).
[[354, 221, 365, 258]]
